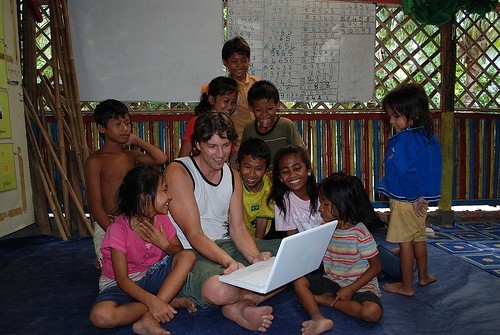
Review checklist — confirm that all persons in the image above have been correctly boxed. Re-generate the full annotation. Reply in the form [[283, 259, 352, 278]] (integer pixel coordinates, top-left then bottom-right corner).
[[383, 83, 444, 296], [90, 166, 198, 335], [221, 37, 259, 139], [241, 80, 306, 179], [179, 77, 238, 158], [266, 144, 403, 283], [85, 99, 168, 271], [292, 170, 383, 335], [162, 111, 288, 332]]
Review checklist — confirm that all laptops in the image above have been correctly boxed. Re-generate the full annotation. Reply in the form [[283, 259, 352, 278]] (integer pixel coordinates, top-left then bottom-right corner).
[[219, 220, 338, 294]]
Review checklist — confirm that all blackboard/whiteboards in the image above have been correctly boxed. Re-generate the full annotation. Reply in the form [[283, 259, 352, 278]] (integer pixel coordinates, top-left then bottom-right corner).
[[227, 0, 376, 103], [68, 0, 223, 102]]
